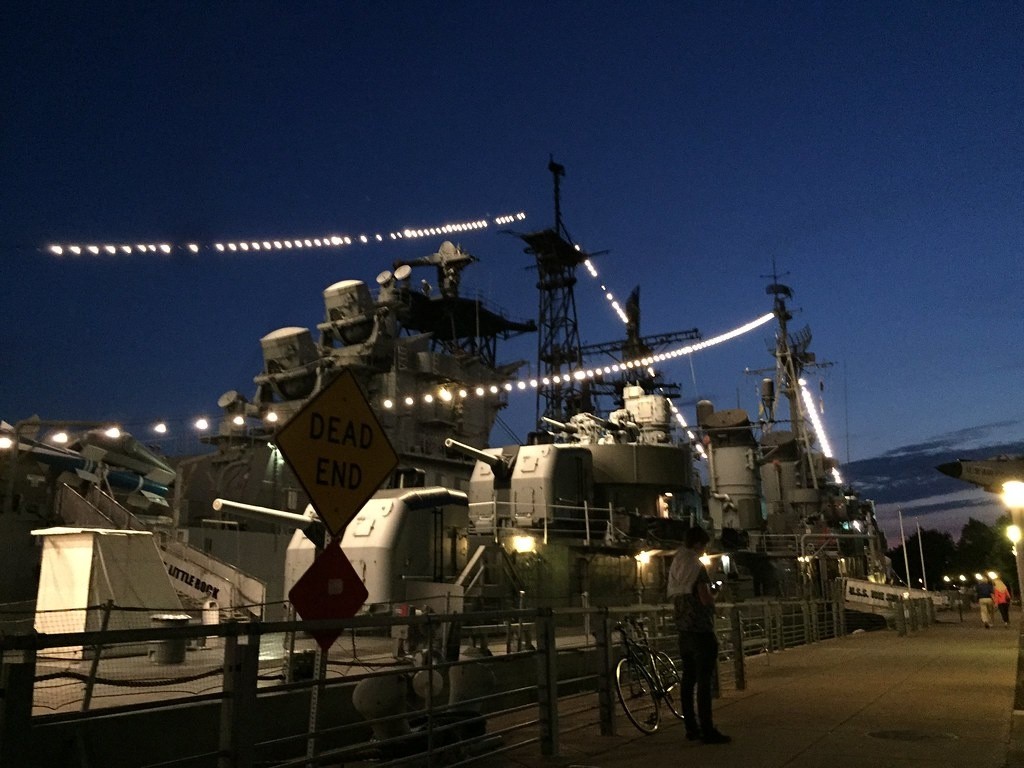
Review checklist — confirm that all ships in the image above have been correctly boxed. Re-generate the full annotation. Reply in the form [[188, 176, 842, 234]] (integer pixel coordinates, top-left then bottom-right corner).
[[0, 150, 878, 768]]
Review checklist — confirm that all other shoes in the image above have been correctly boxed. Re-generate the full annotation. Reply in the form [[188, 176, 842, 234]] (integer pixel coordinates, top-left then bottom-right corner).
[[1001, 619, 1008, 626], [701, 728, 732, 744], [686, 728, 702, 740], [985, 623, 990, 628]]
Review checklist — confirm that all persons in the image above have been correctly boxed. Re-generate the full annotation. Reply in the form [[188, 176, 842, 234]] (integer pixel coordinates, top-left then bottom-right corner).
[[975, 576, 995, 629], [991, 575, 1011, 628], [666, 524, 733, 744]]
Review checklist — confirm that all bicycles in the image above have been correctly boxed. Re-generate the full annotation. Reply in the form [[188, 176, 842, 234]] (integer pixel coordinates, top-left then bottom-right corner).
[[614, 613, 692, 736]]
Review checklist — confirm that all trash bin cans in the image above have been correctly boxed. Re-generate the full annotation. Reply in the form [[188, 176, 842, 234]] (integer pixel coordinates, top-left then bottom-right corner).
[[978, 597, 995, 629], [202, 601, 221, 638]]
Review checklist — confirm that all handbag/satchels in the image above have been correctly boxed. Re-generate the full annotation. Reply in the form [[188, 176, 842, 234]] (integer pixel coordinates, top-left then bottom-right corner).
[[1005, 590, 1011, 601]]
